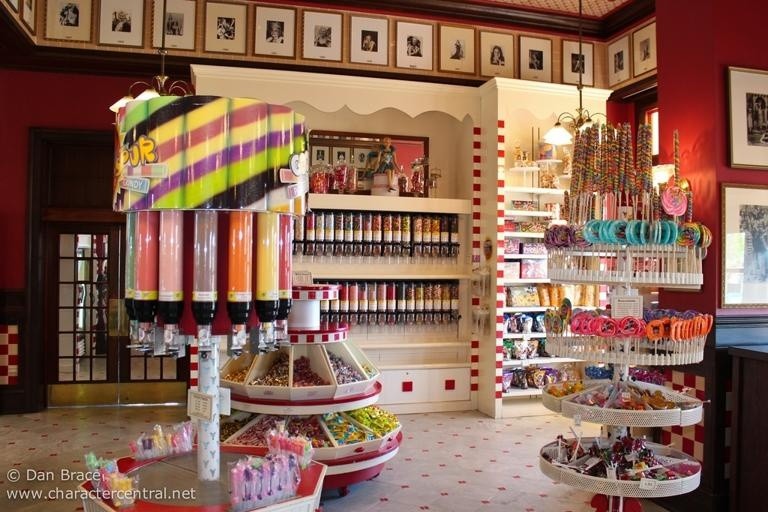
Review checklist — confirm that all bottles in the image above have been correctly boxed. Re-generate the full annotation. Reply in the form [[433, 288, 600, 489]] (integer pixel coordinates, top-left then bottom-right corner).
[[294, 209, 460, 264], [309, 152, 358, 195], [122, 213, 296, 360], [563, 153, 572, 175], [514, 145, 529, 168], [315, 279, 459, 332], [541, 169, 560, 189], [397, 164, 441, 198]]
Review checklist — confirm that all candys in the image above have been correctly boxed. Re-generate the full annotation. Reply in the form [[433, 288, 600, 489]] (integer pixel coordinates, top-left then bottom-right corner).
[[544, 121, 714, 249], [544, 298, 714, 340], [88, 406, 400, 506], [396, 164, 425, 194], [549, 367, 695, 410], [222, 351, 375, 387], [542, 434, 699, 481], [310, 159, 357, 193]]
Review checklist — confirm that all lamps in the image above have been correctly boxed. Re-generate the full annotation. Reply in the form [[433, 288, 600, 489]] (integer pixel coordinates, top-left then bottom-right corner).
[[537, 0, 610, 151]]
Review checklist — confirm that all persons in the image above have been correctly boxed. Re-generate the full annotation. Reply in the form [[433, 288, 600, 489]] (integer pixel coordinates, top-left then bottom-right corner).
[[359, 154, 365, 161], [369, 137, 402, 192], [338, 153, 344, 160], [317, 150, 323, 160]]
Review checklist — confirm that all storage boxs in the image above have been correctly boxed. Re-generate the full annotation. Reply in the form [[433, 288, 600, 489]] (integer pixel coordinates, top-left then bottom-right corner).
[[501, 260, 522, 280], [519, 261, 551, 281], [519, 242, 548, 255], [503, 238, 522, 256]]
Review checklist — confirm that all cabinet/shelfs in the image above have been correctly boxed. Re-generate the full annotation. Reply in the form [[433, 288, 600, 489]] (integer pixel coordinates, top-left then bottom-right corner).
[[293, 189, 482, 418], [501, 182, 602, 400], [721, 339, 768, 512]]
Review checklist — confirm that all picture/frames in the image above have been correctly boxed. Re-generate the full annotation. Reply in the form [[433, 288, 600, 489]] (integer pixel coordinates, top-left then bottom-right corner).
[[715, 179, 767, 311], [723, 64, 768, 172], [6, 1, 659, 91], [308, 143, 374, 173]]
